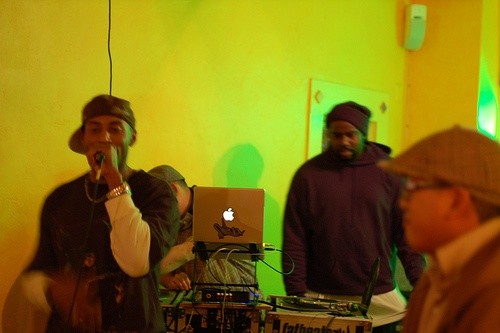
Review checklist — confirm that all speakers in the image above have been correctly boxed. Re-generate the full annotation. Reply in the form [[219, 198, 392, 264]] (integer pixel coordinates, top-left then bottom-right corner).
[[403, 4, 427, 51]]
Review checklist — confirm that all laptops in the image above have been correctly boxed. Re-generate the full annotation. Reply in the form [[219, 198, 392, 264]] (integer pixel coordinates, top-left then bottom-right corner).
[[193, 187, 265, 246]]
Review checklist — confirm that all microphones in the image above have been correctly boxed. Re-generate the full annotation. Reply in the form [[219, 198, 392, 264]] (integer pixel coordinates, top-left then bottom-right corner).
[[95, 152, 105, 162], [361, 257, 379, 315]]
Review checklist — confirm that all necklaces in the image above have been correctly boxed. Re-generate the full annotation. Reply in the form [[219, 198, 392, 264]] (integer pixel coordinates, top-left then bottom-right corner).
[[85, 165, 129, 203]]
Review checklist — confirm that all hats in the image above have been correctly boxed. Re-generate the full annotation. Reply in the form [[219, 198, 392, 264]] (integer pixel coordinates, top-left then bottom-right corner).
[[380, 125, 500, 207], [325, 101, 371, 136], [68, 94, 135, 155], [147, 165, 185, 185]]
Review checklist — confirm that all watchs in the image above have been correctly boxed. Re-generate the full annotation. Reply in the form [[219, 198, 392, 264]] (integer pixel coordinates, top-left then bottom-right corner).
[[105, 181, 132, 200]]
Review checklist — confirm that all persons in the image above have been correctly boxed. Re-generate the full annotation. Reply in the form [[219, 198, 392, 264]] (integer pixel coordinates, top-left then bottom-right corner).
[[395, 127, 500, 333], [282, 103, 426, 332], [16, 95, 180, 332], [147, 164, 262, 301]]
